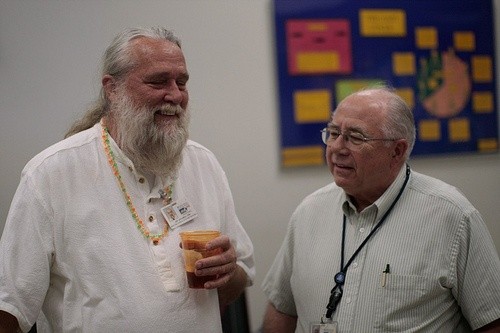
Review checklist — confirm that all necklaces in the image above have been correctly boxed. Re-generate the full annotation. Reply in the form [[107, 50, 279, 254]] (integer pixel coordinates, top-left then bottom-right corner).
[[99, 111, 176, 245]]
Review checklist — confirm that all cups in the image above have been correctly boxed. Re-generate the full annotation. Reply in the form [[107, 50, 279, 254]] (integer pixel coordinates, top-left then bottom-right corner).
[[179, 230, 221, 289]]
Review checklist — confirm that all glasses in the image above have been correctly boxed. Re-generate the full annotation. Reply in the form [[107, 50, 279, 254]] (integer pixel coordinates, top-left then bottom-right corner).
[[320, 127, 397, 151]]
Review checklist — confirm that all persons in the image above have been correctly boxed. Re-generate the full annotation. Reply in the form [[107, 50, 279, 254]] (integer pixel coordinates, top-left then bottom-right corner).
[[0, 24, 256, 333], [260, 88, 500, 333]]
